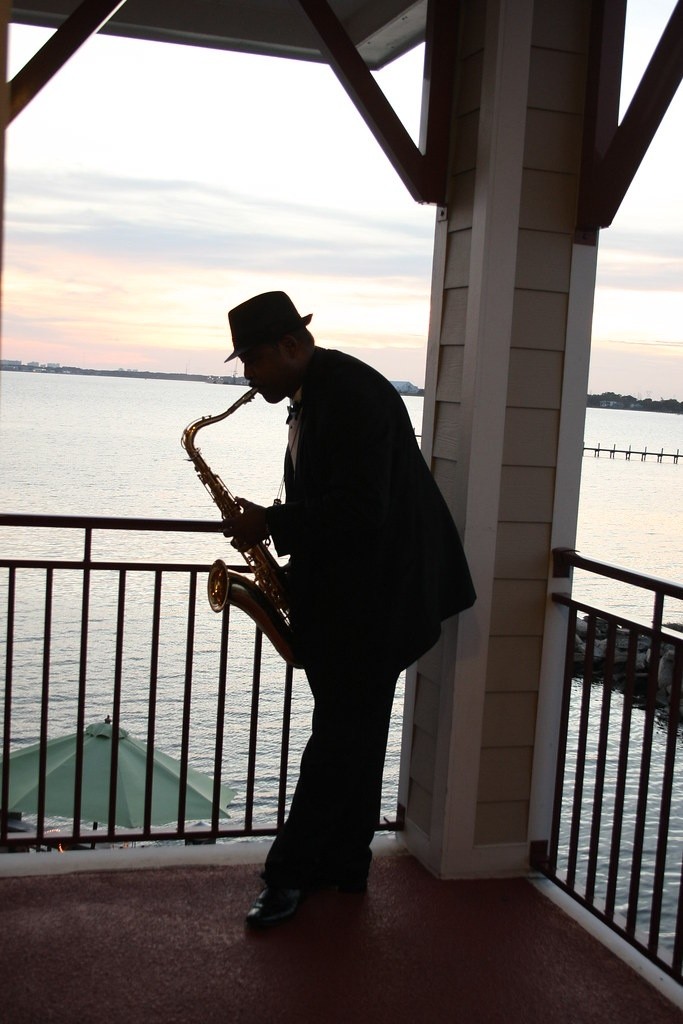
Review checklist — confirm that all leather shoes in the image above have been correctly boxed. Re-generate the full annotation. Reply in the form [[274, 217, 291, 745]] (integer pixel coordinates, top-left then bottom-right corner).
[[245, 879, 303, 925], [306, 864, 368, 895]]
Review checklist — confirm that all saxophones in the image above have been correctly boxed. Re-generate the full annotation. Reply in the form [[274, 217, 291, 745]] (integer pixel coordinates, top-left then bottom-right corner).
[[180, 385, 309, 670]]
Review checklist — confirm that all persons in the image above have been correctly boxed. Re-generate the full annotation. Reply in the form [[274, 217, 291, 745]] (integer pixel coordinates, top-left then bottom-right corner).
[[222, 291, 479, 929]]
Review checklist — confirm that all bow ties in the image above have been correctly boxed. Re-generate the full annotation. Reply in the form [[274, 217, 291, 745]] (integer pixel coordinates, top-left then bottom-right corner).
[[285, 400, 301, 425]]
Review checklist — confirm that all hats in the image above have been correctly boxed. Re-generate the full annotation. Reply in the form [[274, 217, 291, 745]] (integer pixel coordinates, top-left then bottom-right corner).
[[224, 291, 312, 362]]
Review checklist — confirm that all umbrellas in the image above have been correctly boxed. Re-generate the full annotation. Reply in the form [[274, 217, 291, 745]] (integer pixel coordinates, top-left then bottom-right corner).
[[0, 715, 238, 850]]
